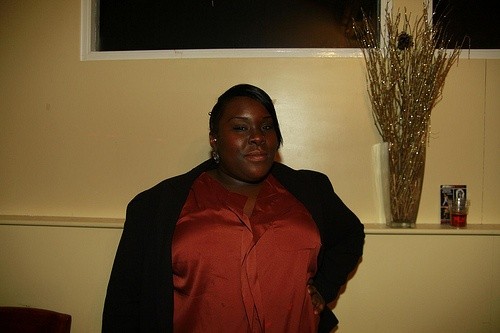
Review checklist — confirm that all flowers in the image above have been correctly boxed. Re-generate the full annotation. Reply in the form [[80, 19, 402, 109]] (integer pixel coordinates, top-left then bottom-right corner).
[[348, 0, 472, 138]]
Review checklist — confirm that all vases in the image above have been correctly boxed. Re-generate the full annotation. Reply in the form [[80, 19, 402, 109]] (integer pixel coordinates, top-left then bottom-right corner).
[[387, 141, 427, 227]]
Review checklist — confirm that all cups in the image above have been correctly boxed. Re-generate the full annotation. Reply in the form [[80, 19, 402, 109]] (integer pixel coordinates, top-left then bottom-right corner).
[[448, 197, 467, 227]]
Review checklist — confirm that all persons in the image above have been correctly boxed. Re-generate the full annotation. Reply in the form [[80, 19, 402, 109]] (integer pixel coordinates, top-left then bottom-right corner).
[[101, 84, 364, 333]]
